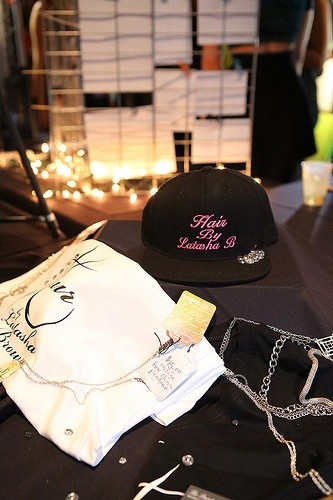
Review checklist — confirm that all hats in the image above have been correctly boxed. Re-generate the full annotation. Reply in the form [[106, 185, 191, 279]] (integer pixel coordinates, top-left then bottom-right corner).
[[141, 168, 278, 283]]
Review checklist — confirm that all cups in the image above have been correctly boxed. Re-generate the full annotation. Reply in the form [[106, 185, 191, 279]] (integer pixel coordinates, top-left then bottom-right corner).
[[300, 161, 333, 207]]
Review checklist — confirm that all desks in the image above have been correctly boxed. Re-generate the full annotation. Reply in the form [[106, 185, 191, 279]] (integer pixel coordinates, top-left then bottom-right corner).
[[0, 178, 333, 500]]
